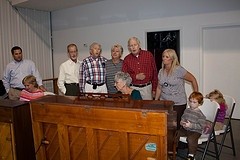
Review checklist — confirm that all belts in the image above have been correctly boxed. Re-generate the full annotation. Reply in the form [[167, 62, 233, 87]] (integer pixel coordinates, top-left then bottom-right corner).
[[14, 87, 24, 91], [65, 83, 79, 86], [86, 81, 106, 86], [129, 82, 151, 88]]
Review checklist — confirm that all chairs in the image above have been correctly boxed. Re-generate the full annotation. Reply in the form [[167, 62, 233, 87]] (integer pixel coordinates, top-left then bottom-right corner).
[[173, 98, 220, 160], [195, 96, 237, 159]]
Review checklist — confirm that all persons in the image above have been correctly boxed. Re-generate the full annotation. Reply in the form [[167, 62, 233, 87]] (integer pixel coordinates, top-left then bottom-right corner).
[[79, 43, 109, 97], [123, 37, 158, 100], [16, 75, 45, 101], [176, 92, 206, 160], [114, 71, 143, 100], [155, 49, 198, 129], [206, 90, 228, 131], [0, 80, 8, 100], [5, 46, 47, 101], [105, 44, 124, 97], [57, 43, 83, 96]]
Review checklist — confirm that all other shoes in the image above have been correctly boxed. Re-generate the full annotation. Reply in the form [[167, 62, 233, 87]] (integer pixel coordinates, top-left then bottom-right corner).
[[186, 153, 195, 160]]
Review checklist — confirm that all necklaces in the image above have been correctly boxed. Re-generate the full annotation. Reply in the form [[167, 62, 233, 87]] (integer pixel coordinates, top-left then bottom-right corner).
[[122, 88, 132, 94], [164, 67, 173, 87]]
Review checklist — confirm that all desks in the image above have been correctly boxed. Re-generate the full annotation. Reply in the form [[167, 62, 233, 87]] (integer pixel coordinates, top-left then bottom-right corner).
[[28, 96, 174, 160], [0, 98, 32, 160]]
